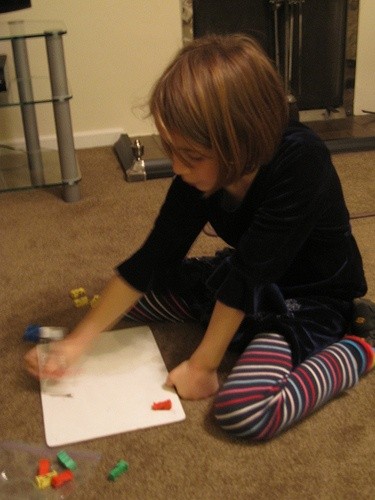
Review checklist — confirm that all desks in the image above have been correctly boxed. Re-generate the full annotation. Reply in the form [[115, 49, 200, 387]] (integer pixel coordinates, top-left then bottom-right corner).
[[0, 20, 83, 202]]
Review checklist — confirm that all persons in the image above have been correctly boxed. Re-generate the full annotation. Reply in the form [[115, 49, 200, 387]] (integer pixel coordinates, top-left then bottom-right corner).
[[22, 34, 375, 442]]
